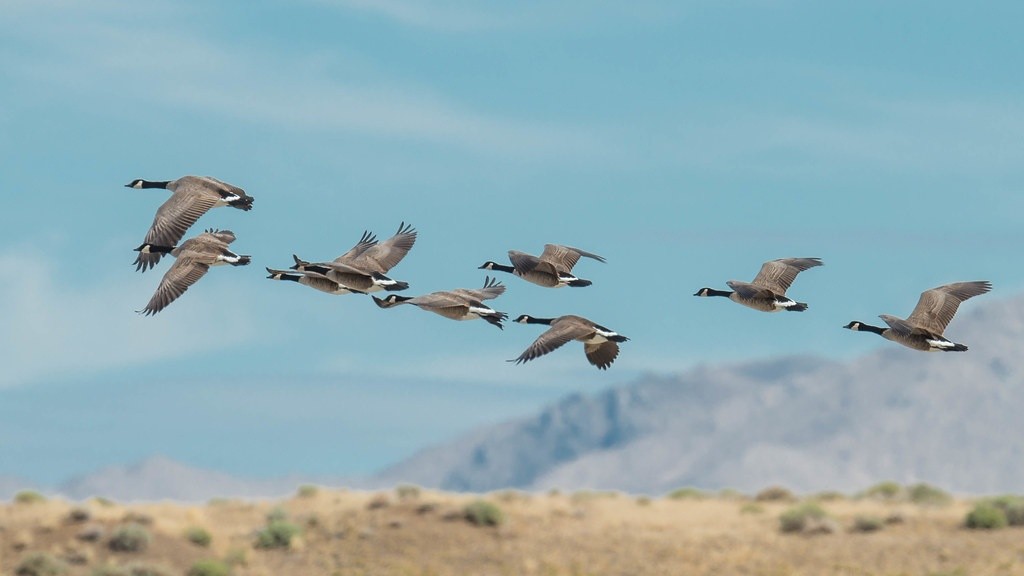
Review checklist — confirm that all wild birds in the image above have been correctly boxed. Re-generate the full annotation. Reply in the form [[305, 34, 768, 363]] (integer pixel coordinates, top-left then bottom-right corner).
[[133, 228, 252, 318], [478, 244, 607, 288], [372, 276, 509, 331], [124, 175, 255, 273], [842, 281, 993, 352], [266, 221, 417, 296], [693, 257, 824, 313], [505, 315, 631, 371]]
